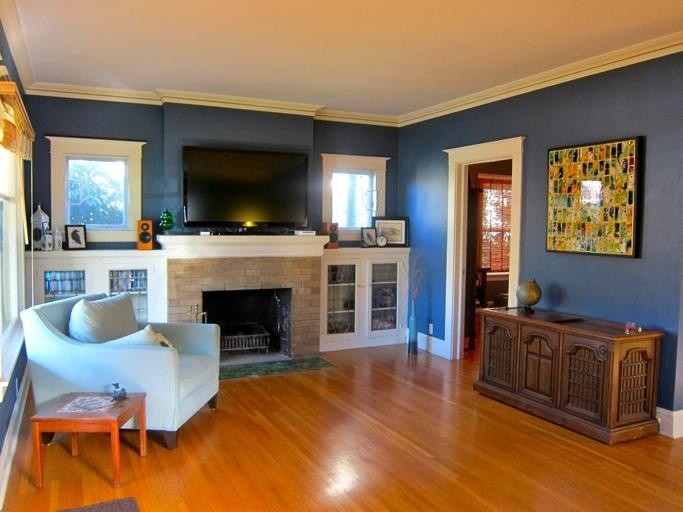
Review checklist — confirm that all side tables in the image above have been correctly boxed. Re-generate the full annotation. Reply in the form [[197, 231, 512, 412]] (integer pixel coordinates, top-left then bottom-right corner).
[[30, 391, 147, 489]]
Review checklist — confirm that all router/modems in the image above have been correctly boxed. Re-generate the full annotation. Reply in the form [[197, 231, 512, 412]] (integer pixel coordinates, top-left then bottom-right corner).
[[288, 230, 316, 236]]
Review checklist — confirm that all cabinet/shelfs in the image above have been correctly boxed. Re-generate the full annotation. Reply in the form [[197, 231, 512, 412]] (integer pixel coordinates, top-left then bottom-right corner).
[[473, 311, 661, 446], [319, 247, 410, 352], [26, 256, 166, 321]]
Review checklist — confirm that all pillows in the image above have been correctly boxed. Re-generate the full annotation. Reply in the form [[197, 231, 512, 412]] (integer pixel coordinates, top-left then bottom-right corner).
[[68, 292, 173, 348]]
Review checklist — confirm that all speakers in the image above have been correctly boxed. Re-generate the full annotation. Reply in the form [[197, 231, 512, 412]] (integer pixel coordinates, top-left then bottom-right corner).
[[137, 219, 153, 250]]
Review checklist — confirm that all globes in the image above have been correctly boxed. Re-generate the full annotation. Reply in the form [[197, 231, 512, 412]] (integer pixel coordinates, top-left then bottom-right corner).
[[514, 278, 542, 315]]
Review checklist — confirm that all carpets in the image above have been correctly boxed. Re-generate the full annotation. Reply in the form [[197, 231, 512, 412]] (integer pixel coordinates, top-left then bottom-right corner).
[[220, 356, 339, 380], [51, 496, 138, 512]]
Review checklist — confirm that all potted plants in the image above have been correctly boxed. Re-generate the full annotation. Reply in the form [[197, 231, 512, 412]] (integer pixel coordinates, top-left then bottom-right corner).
[[397, 246, 430, 355]]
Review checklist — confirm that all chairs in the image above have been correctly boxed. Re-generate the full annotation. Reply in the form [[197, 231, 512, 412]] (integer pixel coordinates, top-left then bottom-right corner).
[[19, 292, 222, 451]]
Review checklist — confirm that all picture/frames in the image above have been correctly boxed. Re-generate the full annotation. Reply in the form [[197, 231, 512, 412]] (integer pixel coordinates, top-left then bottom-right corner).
[[361, 216, 408, 248], [64, 225, 87, 250]]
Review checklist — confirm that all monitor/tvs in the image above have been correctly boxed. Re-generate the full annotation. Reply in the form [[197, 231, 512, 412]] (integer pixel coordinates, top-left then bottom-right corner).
[[179, 144, 308, 236]]
